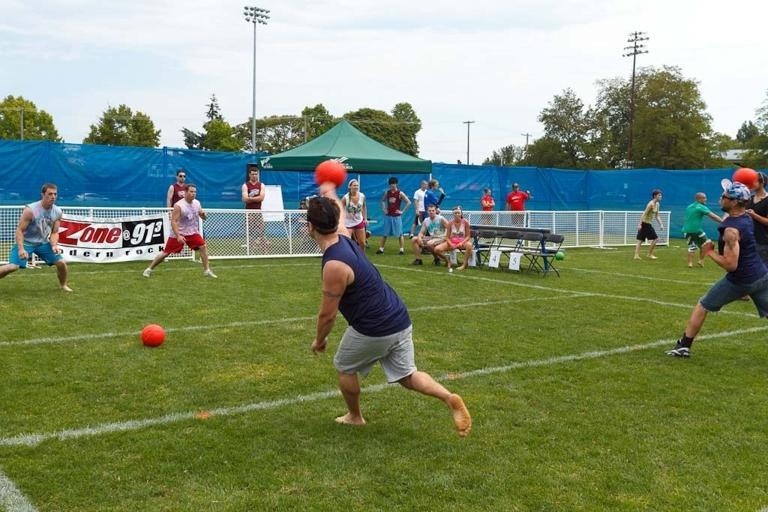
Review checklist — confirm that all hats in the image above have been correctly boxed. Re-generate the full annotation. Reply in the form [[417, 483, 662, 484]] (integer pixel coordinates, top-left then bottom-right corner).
[[725, 181, 751, 203]]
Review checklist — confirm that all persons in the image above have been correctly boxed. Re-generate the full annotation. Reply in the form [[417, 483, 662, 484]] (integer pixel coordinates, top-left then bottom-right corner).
[[433, 206, 474, 270], [142, 183, 219, 279], [662, 167, 768, 357], [480, 187, 495, 224], [165, 170, 188, 208], [634, 189, 664, 260], [504, 182, 530, 224], [412, 204, 447, 266], [376, 176, 411, 254], [306, 159, 472, 438], [342, 179, 367, 254], [0, 182, 73, 294], [294, 199, 309, 249], [410, 180, 428, 239], [423, 179, 446, 220], [239, 169, 271, 248]]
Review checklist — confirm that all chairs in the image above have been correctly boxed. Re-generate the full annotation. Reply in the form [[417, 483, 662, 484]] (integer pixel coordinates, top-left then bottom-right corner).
[[421, 224, 565, 278]]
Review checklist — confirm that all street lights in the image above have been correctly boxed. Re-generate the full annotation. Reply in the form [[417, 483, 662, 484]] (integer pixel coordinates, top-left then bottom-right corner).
[[243, 5, 271, 156], [619, 30, 651, 161]]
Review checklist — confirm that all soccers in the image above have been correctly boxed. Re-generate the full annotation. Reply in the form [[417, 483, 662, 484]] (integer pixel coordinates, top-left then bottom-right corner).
[[555, 252, 565, 260], [730, 166, 757, 189], [142, 325, 165, 347], [316, 160, 347, 187]]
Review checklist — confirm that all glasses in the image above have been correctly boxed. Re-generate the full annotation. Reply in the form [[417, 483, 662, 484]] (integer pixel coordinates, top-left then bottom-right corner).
[[720, 193, 735, 201]]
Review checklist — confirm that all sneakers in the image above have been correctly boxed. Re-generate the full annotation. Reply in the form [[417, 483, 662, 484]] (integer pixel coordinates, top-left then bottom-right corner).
[[142, 267, 153, 278], [202, 268, 218, 279], [375, 248, 442, 267], [664, 340, 691, 359]]
